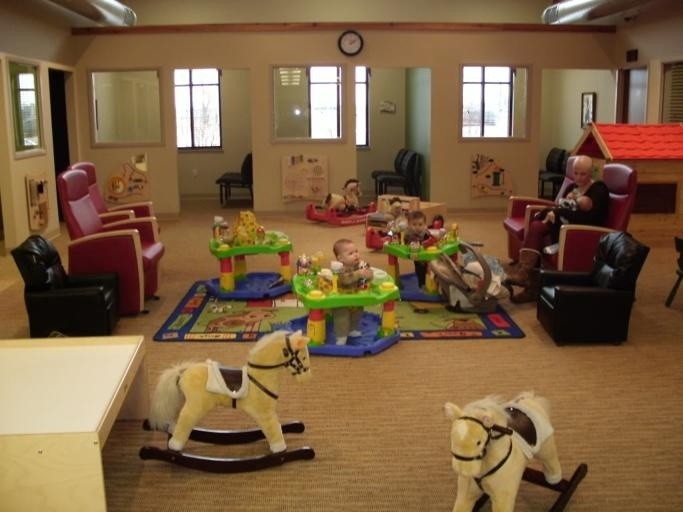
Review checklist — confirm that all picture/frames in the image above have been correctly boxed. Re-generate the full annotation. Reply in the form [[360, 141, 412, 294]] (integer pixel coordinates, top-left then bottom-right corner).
[[581, 92, 596, 129]]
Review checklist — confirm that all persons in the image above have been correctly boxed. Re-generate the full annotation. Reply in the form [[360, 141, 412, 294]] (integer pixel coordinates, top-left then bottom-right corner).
[[329, 239, 373, 347], [501, 155, 611, 308], [401, 210, 431, 290], [534, 193, 592, 226]]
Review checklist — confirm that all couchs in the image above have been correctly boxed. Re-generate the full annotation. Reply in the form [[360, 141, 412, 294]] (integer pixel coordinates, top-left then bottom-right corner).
[[541, 164, 638, 271], [503, 156, 579, 266], [10, 234, 118, 338], [67, 161, 153, 223], [57, 169, 166, 314], [536, 231, 650, 347]]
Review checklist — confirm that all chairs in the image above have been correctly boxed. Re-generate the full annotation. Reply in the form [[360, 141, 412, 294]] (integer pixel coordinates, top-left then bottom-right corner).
[[215, 153, 253, 206], [540, 148, 566, 200], [664, 236, 683, 306], [377, 151, 417, 196], [372, 148, 408, 194], [537, 147, 559, 194]]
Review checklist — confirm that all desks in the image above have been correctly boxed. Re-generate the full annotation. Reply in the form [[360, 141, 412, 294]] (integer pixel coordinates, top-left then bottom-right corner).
[[0, 336, 149, 511]]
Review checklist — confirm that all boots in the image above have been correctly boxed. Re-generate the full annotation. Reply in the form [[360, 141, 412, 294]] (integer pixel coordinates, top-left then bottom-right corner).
[[504, 247, 542, 281], [509, 268, 540, 303]]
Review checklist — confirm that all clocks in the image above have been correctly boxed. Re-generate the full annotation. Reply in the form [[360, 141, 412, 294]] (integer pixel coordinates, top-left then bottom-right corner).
[[338, 30, 363, 57]]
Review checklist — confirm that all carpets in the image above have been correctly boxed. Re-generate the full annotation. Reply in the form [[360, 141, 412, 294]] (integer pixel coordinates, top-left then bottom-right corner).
[[153, 279, 527, 343]]
